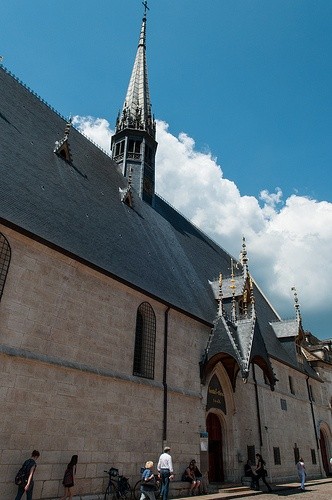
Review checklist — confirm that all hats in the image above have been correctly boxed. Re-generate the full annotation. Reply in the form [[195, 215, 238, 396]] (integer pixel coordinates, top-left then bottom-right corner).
[[164, 447, 170, 450], [145, 461, 153, 468]]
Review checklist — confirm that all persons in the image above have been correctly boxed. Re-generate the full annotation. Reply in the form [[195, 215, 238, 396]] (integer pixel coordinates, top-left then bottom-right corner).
[[297, 458, 306, 490], [182, 459, 208, 496], [15, 450, 40, 500], [140, 447, 174, 500], [244, 454, 273, 493], [60, 455, 78, 500]]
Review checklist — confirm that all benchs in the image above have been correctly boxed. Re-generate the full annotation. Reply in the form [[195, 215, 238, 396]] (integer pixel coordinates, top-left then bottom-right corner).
[[168, 481, 200, 498], [241, 476, 252, 486]]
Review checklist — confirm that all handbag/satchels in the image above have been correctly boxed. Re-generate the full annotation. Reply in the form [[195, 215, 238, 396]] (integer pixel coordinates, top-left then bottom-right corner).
[[141, 479, 160, 492], [108, 467, 118, 477]]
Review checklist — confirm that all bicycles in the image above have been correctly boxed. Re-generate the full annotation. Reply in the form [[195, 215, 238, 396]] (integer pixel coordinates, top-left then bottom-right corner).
[[133, 468, 163, 500], [104, 467, 133, 500]]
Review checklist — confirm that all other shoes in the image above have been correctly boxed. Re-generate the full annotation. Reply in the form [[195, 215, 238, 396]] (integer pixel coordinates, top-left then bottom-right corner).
[[199, 491, 203, 495], [193, 490, 196, 496], [188, 489, 192, 497], [298, 487, 305, 493], [255, 488, 259, 490], [204, 489, 208, 494], [267, 488, 272, 492]]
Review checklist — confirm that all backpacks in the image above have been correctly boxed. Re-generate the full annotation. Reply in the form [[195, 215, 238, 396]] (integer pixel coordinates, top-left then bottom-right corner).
[[15, 460, 33, 486]]
